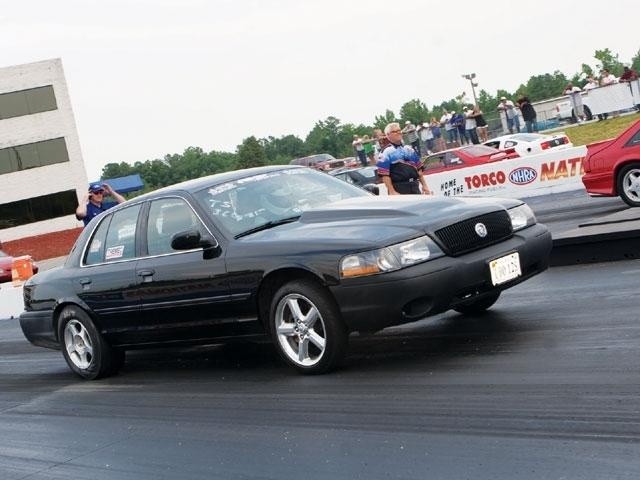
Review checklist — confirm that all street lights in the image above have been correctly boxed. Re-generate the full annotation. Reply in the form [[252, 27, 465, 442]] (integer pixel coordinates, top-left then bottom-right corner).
[[463, 73, 480, 112]]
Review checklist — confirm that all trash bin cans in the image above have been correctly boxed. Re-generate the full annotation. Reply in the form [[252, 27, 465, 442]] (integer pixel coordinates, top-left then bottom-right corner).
[[11, 254, 34, 282]]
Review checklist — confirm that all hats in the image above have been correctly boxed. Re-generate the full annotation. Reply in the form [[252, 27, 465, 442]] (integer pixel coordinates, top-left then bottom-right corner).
[[88, 185, 104, 193]]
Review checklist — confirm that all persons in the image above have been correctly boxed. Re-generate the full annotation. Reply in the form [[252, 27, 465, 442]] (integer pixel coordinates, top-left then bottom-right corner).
[[496, 96, 521, 134], [582, 74, 609, 122], [618, 66, 640, 113], [561, 80, 587, 123], [599, 68, 620, 119], [75, 181, 127, 226], [351, 104, 488, 167], [375, 121, 431, 196], [515, 92, 539, 133]]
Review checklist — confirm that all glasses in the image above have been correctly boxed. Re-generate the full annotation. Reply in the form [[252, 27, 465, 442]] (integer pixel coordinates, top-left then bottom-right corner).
[[94, 191, 103, 195]]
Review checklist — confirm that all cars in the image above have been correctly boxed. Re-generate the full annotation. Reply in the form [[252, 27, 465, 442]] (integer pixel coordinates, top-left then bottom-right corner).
[[581, 117, 640, 208], [0, 248, 38, 283], [20, 164, 554, 381], [420, 142, 520, 176], [289, 153, 380, 184], [482, 132, 574, 158]]
